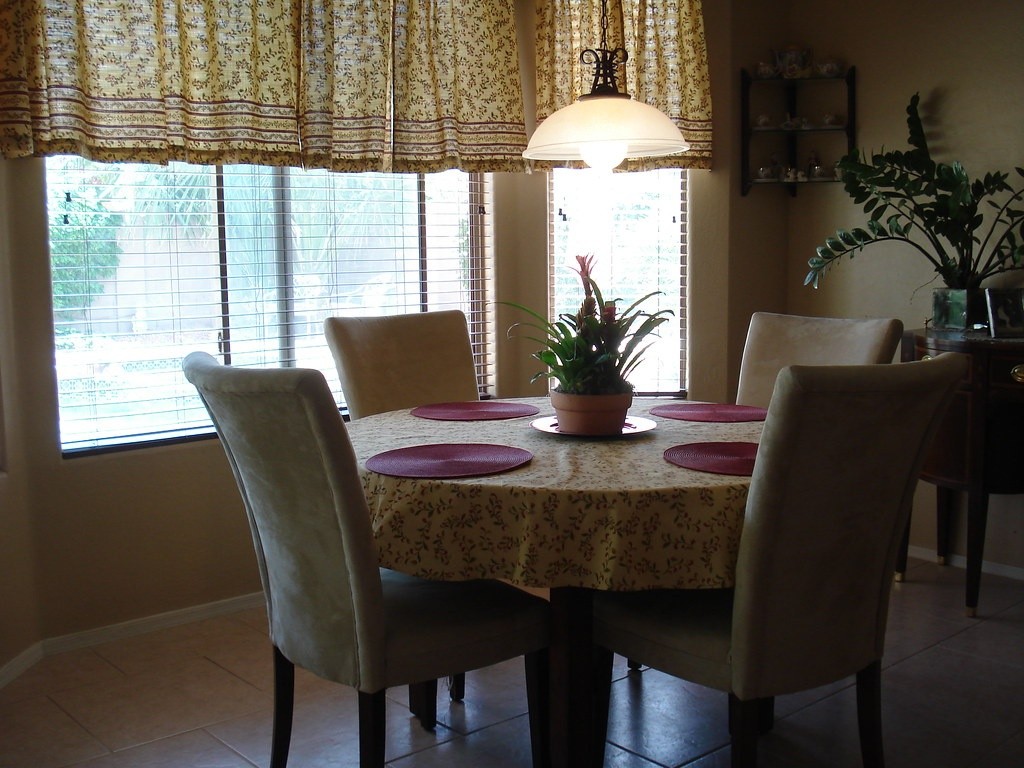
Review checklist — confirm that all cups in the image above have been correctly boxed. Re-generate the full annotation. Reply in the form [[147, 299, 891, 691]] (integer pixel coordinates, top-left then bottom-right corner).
[[932, 287, 967, 332]]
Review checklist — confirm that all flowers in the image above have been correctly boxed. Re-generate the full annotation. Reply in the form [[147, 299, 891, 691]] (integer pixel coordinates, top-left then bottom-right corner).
[[485, 253, 676, 396]]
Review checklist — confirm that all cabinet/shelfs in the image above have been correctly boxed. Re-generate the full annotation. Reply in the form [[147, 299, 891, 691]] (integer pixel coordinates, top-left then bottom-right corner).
[[740, 66, 856, 196], [895, 328, 1023, 617]]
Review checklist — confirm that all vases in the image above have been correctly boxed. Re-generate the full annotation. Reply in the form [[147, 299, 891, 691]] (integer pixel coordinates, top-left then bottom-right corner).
[[549, 389, 633, 435]]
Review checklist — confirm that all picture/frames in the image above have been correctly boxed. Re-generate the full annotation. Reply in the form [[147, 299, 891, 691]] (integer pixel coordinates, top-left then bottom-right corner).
[[985, 288, 1024, 339]]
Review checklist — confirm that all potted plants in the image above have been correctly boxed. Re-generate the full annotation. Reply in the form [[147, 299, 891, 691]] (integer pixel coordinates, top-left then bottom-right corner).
[[803, 92, 1024, 334]]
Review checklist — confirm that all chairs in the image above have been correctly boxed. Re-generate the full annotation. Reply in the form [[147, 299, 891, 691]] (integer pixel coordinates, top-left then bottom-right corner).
[[593, 351, 970, 768], [737, 310, 904, 409], [182, 310, 533, 768]]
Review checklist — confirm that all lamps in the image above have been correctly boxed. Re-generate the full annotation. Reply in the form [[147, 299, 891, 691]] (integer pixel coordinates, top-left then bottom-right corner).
[[522, 0, 691, 172]]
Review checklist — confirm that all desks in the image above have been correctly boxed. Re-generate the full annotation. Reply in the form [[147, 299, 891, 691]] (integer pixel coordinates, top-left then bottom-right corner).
[[341, 396, 769, 768]]
